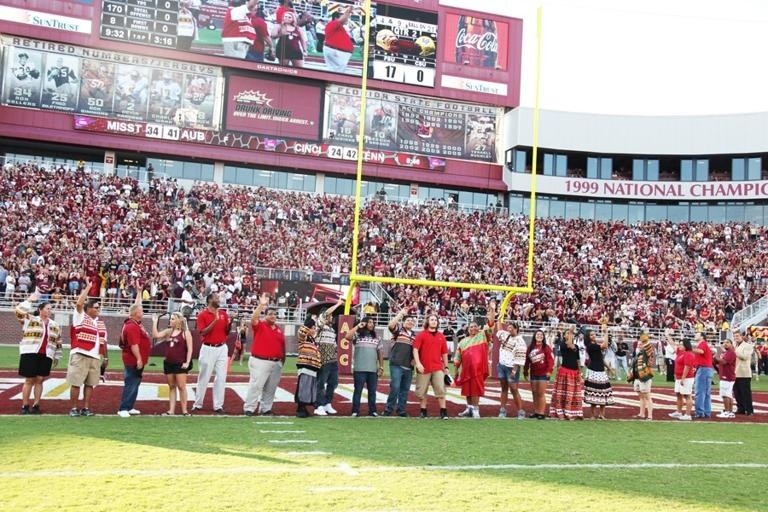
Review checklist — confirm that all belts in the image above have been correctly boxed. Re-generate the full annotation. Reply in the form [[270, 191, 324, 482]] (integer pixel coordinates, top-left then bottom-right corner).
[[204, 341, 225, 347], [251, 353, 281, 361], [325, 45, 353, 55]]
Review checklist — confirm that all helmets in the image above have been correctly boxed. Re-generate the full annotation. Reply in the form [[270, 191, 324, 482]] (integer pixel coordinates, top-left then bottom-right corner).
[[414, 36, 436, 57], [375, 29, 400, 52]]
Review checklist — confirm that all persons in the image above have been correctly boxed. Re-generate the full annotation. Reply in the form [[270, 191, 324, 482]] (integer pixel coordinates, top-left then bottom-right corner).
[[0, 157, 767, 423], [14, 0, 493, 161]]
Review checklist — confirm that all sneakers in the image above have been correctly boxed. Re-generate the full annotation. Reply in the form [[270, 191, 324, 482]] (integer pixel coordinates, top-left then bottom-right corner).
[[668, 411, 682, 418], [677, 414, 692, 420], [715, 411, 735, 419]]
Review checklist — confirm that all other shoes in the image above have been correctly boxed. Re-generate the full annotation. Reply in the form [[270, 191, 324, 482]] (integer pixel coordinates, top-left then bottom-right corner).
[[20, 406, 31, 414], [214, 409, 224, 413], [647, 414, 652, 419], [382, 411, 392, 416], [472, 407, 480, 420], [184, 413, 189, 416], [69, 408, 80, 417], [190, 407, 200, 415], [458, 407, 472, 417], [632, 414, 645, 419], [734, 410, 746, 415], [692, 412, 705, 420], [498, 407, 507, 419], [296, 406, 309, 418], [31, 406, 42, 414], [128, 408, 141, 414], [746, 408, 753, 416], [263, 410, 275, 416], [704, 413, 712, 419], [162, 411, 174, 416], [80, 408, 94, 416], [439, 408, 449, 420], [246, 411, 253, 417], [517, 409, 526, 420], [420, 408, 428, 419], [324, 403, 337, 414], [368, 411, 378, 417], [314, 406, 328, 416], [117, 410, 130, 418], [528, 411, 607, 421], [397, 411, 407, 417], [352, 412, 359, 417]]
